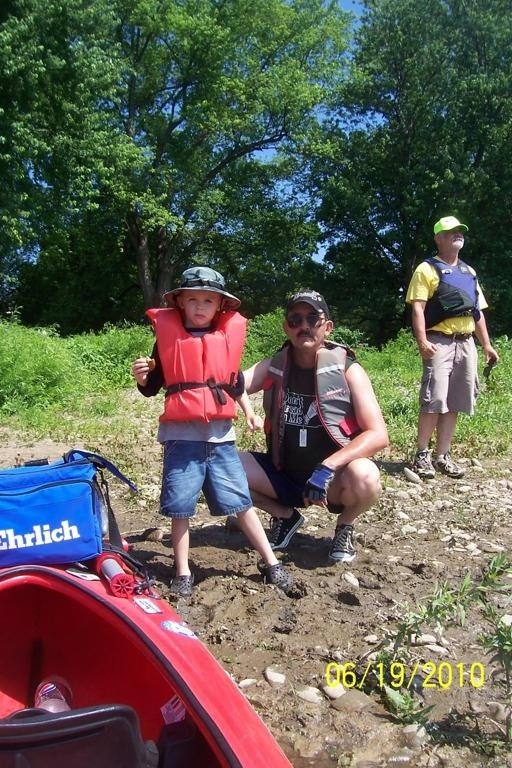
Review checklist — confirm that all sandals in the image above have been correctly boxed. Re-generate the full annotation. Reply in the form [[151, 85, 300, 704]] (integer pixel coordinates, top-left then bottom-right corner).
[[256, 557, 306, 599], [170, 569, 195, 600]]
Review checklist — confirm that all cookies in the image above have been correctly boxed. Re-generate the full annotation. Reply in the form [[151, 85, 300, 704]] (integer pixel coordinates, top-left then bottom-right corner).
[[140, 355, 155, 371]]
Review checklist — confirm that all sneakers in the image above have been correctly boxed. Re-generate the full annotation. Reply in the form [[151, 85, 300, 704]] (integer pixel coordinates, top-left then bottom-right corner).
[[328, 523, 357, 563], [434, 454, 465, 478], [267, 509, 304, 552], [412, 450, 437, 479]]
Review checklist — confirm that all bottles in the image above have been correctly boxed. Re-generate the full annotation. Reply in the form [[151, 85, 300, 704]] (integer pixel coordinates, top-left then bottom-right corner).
[[34, 673, 73, 713]]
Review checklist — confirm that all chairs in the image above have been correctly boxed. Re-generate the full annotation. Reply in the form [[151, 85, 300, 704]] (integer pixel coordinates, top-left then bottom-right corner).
[[0, 703, 150, 767]]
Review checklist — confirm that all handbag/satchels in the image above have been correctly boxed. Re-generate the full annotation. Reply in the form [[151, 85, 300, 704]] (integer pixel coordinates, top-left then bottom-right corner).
[[0, 448, 105, 570]]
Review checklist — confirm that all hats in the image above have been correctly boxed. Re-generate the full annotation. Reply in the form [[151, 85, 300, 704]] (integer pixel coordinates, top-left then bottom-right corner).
[[161, 267, 241, 313], [434, 215, 468, 233], [286, 288, 331, 320]]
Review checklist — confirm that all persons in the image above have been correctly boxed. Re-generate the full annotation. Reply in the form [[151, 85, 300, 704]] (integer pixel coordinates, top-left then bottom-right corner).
[[131, 265, 309, 601], [234, 289, 390, 564], [405, 214, 501, 478]]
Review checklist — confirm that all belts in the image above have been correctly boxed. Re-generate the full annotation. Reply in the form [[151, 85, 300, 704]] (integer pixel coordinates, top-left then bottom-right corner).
[[425, 327, 475, 341]]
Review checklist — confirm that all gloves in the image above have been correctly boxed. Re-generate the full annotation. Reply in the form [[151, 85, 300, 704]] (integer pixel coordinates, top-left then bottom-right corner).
[[303, 463, 334, 503]]
